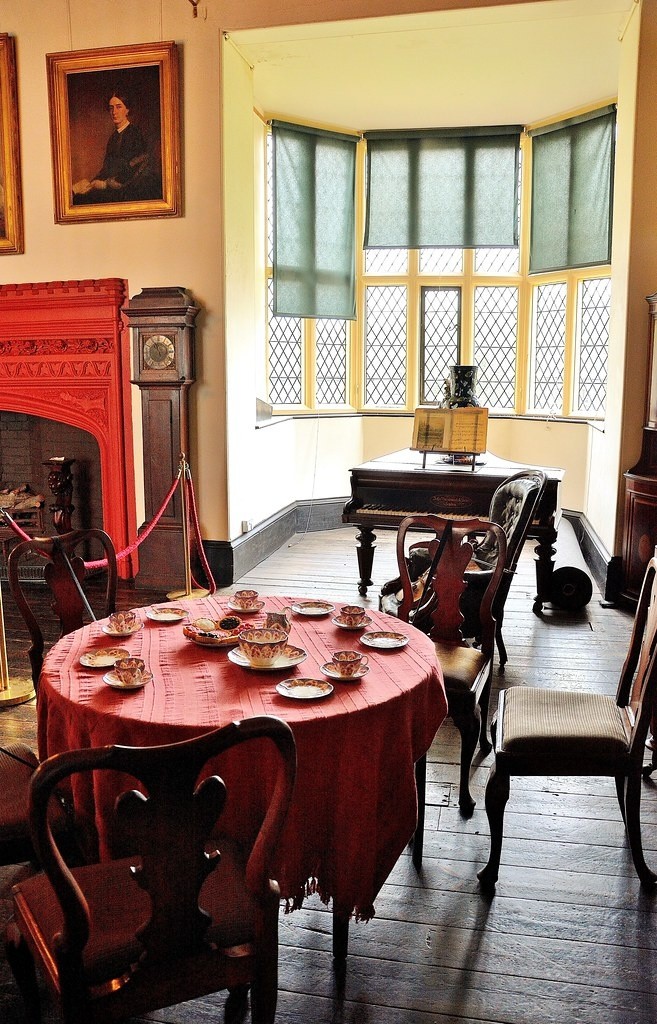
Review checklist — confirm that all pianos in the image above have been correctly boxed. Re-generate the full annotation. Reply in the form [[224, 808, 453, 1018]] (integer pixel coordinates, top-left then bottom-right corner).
[[342, 445, 564, 612]]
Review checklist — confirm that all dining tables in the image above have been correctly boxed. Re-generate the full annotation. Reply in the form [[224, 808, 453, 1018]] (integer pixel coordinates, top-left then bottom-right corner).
[[35, 590, 449, 963]]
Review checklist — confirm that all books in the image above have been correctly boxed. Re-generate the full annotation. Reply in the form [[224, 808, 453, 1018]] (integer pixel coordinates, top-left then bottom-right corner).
[[411, 406, 487, 453]]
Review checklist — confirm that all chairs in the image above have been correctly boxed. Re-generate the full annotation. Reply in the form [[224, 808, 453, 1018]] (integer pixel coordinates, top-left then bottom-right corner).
[[11, 716, 297, 1024], [378, 469, 548, 665], [7, 529, 116, 694], [476, 553, 657, 887], [397, 514, 505, 812]]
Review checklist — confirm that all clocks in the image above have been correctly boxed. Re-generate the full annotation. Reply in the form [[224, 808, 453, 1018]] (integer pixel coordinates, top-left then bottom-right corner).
[[119, 285, 202, 596]]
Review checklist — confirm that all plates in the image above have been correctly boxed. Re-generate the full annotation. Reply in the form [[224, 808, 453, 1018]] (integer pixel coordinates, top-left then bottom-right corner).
[[101, 622, 144, 636], [291, 601, 335, 615], [80, 647, 131, 667], [228, 600, 265, 612], [227, 644, 308, 670], [359, 631, 409, 648], [189, 637, 239, 646], [275, 678, 334, 699], [145, 608, 190, 622], [332, 616, 373, 629], [319, 662, 370, 680], [102, 670, 154, 688]]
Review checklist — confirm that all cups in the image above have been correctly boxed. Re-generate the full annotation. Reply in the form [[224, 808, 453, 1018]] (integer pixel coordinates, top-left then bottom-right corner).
[[332, 650, 369, 676], [109, 611, 136, 632], [114, 658, 145, 684], [340, 605, 365, 626], [229, 590, 259, 608]]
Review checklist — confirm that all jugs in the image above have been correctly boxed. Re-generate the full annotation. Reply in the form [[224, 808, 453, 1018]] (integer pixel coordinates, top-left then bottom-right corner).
[[263, 607, 291, 635]]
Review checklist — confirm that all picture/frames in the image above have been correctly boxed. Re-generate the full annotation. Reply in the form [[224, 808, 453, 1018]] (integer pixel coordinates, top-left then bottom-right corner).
[[45, 39, 182, 226], [0, 32, 25, 256]]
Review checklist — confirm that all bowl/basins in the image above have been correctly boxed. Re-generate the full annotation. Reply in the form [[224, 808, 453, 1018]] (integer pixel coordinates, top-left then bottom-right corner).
[[238, 628, 289, 665]]
